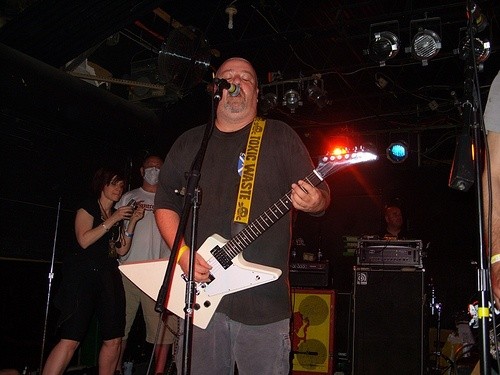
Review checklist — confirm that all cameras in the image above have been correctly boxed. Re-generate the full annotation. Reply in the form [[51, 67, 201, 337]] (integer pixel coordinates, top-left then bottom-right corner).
[[127, 198, 137, 208]]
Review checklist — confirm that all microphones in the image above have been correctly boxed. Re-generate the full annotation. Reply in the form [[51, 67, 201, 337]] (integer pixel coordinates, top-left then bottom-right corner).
[[214, 77, 240, 97]]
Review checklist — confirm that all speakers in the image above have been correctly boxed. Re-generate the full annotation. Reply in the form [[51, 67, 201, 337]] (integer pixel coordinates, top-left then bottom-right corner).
[[335, 266, 427, 375], [289, 288, 338, 375]]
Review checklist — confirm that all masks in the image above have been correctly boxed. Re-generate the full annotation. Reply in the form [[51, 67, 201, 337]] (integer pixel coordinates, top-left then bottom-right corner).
[[143, 167, 160, 186]]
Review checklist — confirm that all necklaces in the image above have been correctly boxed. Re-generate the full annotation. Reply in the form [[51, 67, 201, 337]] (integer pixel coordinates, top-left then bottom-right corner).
[[99, 199, 112, 218]]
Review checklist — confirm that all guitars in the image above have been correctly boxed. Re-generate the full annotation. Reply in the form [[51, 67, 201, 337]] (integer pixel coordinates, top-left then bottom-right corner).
[[117, 145, 379, 330]]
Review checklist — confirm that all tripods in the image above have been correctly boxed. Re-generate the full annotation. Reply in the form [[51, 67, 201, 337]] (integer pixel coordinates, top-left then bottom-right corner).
[[427, 287, 454, 375]]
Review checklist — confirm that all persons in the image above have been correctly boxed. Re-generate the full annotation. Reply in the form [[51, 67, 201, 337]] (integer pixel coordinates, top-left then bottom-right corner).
[[482, 69, 500, 311], [114, 154, 179, 375], [372, 206, 413, 241], [154, 57, 331, 375], [43, 167, 146, 375]]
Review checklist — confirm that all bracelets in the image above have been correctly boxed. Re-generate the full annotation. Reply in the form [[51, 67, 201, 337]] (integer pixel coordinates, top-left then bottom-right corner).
[[491, 254, 500, 264], [125, 231, 134, 238], [177, 245, 189, 265], [102, 222, 109, 231]]
